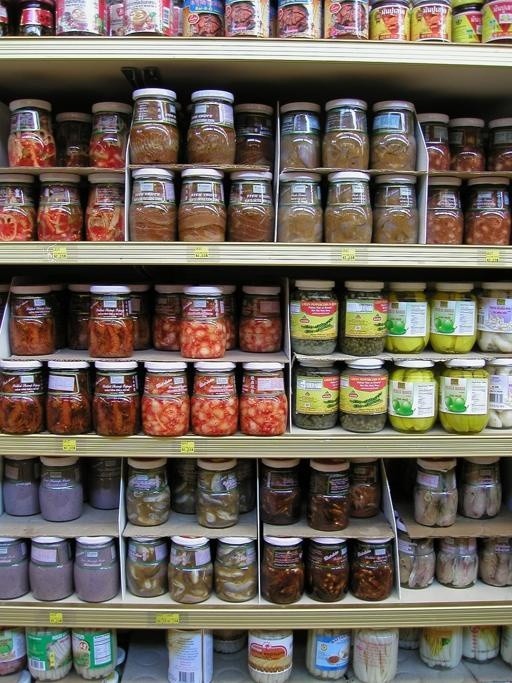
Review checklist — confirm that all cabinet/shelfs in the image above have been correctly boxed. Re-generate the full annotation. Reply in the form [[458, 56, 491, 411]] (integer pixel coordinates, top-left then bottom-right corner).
[[0, 32, 512, 636]]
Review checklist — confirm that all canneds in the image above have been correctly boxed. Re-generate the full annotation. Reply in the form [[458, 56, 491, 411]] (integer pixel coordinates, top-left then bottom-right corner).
[[0, 455, 512, 603], [0, 88, 512, 245], [0, 0, 512, 45], [167, 625, 512, 682], [0, 279, 512, 436], [0, 626, 127, 683]]
